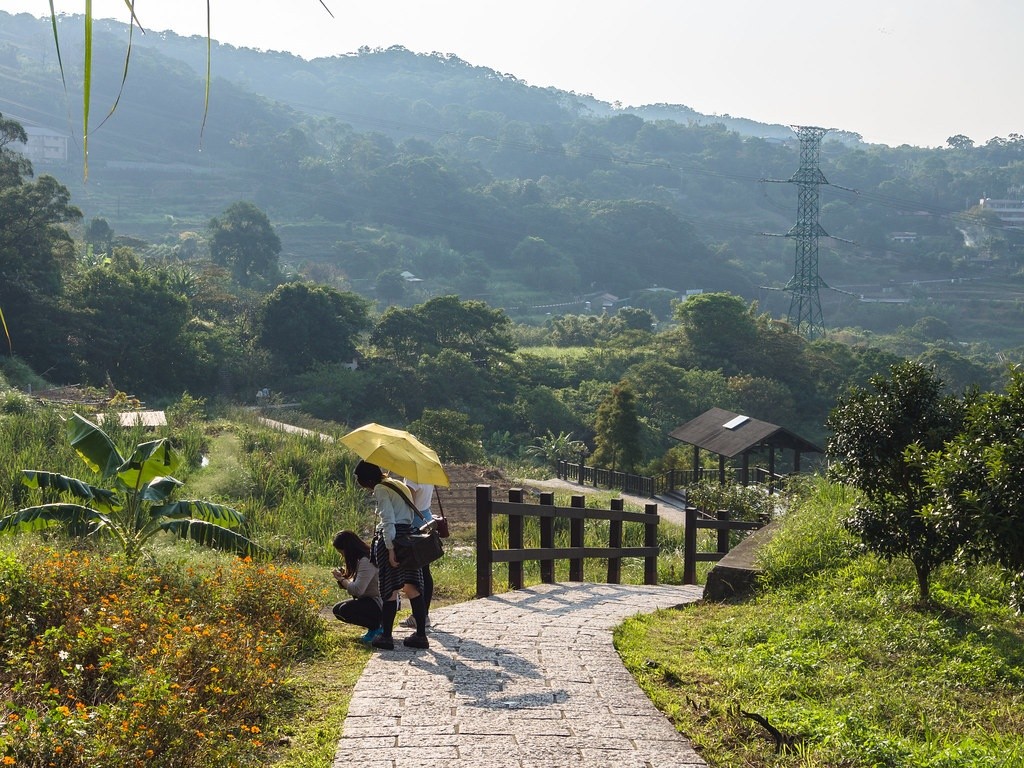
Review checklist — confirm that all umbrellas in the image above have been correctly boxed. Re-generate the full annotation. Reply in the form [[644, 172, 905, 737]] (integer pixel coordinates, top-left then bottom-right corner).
[[338, 422, 450, 488]]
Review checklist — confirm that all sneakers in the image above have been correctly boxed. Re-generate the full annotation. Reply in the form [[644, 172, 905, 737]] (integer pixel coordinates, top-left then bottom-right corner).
[[399, 614, 430, 627], [372, 634, 394, 649], [363, 628, 384, 642], [404, 633, 429, 648]]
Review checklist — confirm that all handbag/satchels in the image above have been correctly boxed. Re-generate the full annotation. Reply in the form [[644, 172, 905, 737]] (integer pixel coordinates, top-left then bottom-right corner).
[[393, 528, 444, 569], [432, 514, 450, 537]]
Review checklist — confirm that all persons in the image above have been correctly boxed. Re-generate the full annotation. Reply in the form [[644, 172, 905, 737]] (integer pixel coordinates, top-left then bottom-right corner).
[[354, 459, 429, 650], [331, 530, 383, 643], [401, 478, 434, 626], [256, 386, 270, 405]]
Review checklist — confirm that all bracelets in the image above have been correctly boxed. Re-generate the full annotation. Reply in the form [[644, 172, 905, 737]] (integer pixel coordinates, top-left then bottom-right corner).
[[340, 578, 345, 583]]
